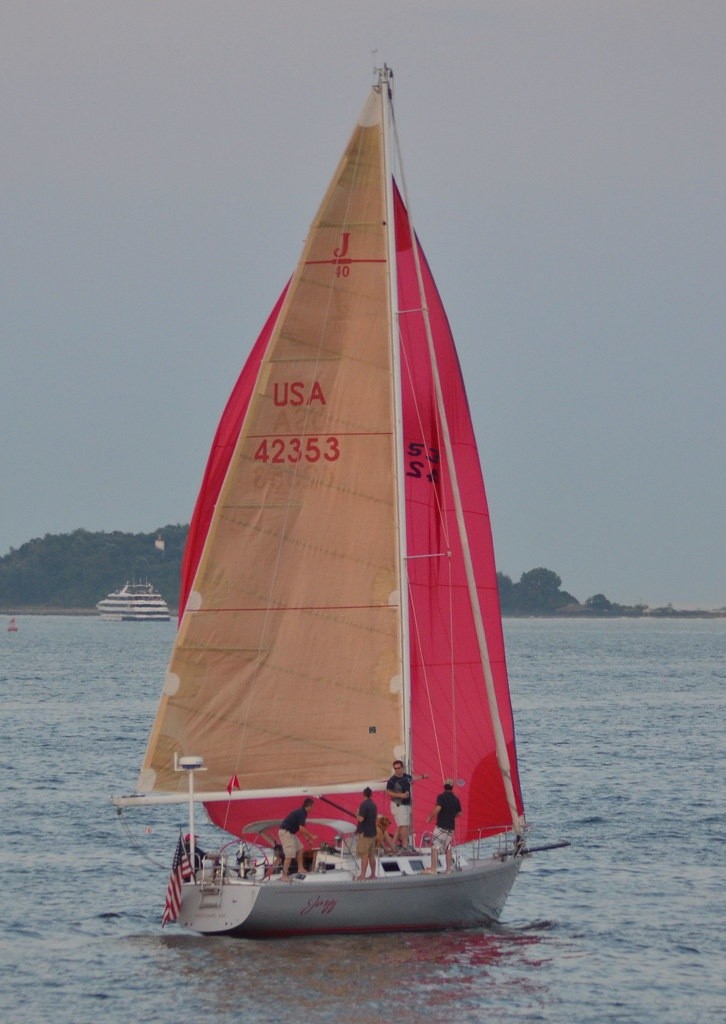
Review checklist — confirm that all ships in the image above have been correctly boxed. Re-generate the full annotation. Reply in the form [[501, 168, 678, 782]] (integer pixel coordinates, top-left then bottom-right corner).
[[96, 577, 170, 623]]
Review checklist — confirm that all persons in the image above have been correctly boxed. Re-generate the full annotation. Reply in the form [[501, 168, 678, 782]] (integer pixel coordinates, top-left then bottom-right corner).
[[385, 760, 428, 850], [182, 833, 207, 882], [356, 786, 377, 880], [424, 778, 462, 875], [270, 798, 317, 882]]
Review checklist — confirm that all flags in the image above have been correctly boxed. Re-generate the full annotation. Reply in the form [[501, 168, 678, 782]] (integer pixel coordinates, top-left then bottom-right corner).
[[161, 832, 193, 928]]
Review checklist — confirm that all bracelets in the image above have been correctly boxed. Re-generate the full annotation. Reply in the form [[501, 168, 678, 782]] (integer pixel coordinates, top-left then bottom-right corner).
[[422, 775, 424, 779]]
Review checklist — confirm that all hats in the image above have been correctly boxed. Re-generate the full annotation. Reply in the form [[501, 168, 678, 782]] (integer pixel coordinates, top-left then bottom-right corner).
[[185, 833, 199, 840]]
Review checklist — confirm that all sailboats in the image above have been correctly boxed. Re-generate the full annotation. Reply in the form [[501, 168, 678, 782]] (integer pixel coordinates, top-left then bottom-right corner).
[[110, 64, 534, 941]]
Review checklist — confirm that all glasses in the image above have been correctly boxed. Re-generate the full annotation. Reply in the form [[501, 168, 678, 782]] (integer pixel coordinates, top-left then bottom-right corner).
[[394, 767, 401, 769]]
